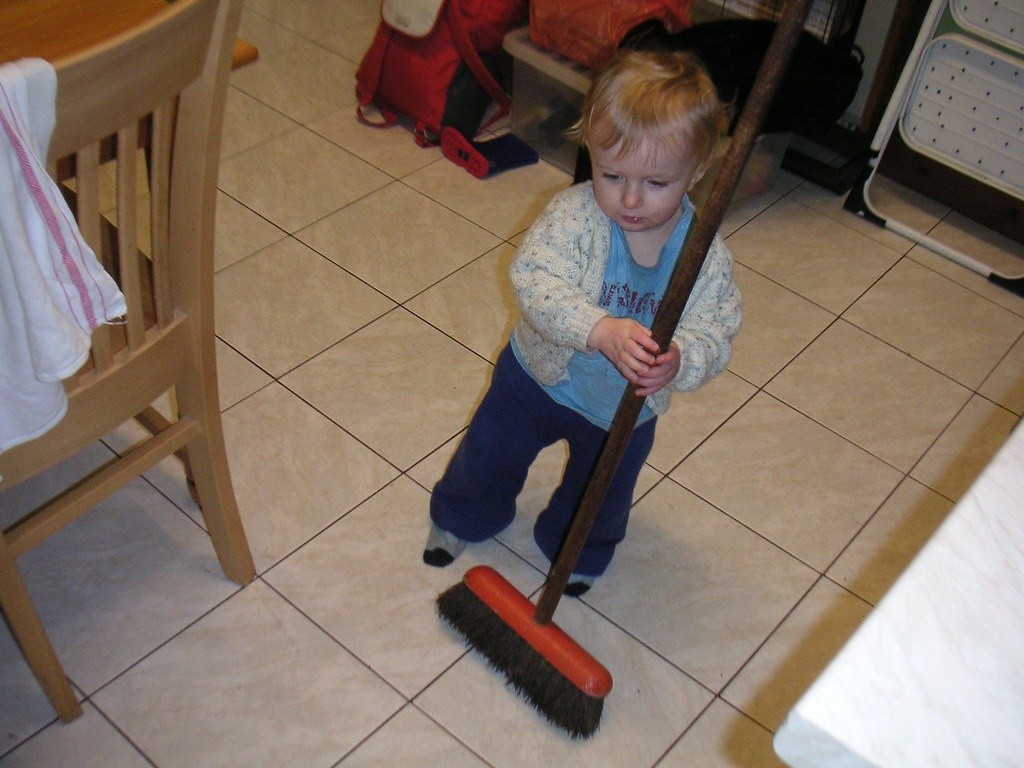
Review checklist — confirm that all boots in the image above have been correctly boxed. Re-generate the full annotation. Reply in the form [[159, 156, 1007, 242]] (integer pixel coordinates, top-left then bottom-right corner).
[[439, 126, 538, 178]]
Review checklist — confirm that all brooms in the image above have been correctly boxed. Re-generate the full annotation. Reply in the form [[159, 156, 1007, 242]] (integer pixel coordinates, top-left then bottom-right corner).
[[433, 0, 815, 737]]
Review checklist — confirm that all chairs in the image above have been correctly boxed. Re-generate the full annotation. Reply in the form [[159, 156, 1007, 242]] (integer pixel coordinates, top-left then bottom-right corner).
[[0, 0, 257, 723]]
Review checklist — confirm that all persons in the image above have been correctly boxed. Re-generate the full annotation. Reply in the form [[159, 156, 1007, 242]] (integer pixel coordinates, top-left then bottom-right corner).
[[420, 49, 744, 599]]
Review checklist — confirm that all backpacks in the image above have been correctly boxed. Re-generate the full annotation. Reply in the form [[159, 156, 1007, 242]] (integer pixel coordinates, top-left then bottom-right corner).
[[355, 0, 530, 148]]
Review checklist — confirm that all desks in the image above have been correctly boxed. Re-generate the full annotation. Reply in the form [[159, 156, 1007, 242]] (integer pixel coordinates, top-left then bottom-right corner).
[[0, 0, 262, 513]]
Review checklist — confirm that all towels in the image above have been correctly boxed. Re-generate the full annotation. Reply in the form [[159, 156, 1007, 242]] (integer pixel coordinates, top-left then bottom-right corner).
[[1, 57, 129, 453]]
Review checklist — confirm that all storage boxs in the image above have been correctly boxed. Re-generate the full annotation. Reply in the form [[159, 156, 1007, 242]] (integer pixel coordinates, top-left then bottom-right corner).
[[502, 26, 795, 223]]
